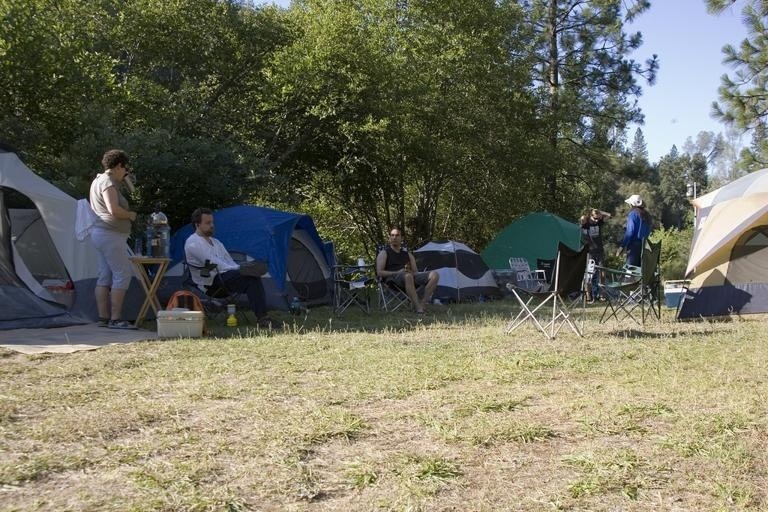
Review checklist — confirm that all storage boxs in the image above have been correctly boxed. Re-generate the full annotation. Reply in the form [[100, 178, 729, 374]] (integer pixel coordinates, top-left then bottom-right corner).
[[663, 280, 691, 307], [155, 307, 205, 340]]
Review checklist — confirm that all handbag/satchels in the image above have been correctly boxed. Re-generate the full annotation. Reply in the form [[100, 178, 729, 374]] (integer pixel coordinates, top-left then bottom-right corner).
[[167, 290, 203, 311]]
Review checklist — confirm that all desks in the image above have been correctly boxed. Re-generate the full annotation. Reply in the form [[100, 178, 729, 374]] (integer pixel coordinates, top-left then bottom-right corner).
[[127, 258, 174, 330]]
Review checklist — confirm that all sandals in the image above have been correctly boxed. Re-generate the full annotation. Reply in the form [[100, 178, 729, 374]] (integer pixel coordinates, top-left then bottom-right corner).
[[108, 319, 137, 330], [416, 307, 425, 314], [97, 318, 110, 326]]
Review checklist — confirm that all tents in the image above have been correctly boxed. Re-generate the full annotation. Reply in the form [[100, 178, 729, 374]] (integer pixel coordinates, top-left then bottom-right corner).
[[480, 213, 587, 301], [1, 150, 159, 328], [676, 170, 768, 319], [408, 238, 504, 303], [149, 203, 340, 315]]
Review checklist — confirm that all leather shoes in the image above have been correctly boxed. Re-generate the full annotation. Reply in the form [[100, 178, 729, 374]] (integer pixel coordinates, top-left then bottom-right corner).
[[240, 263, 267, 277], [259, 318, 283, 330]]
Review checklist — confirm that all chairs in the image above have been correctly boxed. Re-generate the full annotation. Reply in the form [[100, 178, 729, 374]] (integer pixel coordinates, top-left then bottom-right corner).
[[374, 259, 427, 313], [508, 255, 548, 288], [598, 236, 664, 325], [535, 256, 557, 282], [182, 259, 252, 325], [503, 241, 590, 342], [332, 263, 374, 318], [581, 259, 595, 304]]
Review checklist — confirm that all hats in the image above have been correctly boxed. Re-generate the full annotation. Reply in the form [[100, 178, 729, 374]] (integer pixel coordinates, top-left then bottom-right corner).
[[624, 195, 645, 207]]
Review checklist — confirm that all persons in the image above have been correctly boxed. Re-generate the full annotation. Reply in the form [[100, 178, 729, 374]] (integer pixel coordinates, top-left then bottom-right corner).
[[89, 149, 136, 330], [616, 195, 653, 275], [377, 226, 439, 315], [578, 208, 611, 302], [184, 208, 280, 330]]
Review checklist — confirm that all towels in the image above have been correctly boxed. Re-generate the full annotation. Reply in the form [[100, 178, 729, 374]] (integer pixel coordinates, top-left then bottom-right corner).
[[75, 198, 96, 241]]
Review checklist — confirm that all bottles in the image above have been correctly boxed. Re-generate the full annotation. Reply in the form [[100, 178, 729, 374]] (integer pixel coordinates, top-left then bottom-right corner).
[[357, 258, 366, 272], [291, 296, 301, 316]]
